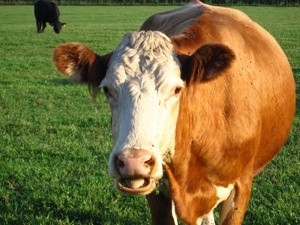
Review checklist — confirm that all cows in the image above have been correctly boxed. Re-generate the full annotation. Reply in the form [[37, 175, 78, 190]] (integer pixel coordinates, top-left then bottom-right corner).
[[33, 1, 66, 34], [52, 1, 300, 225]]
